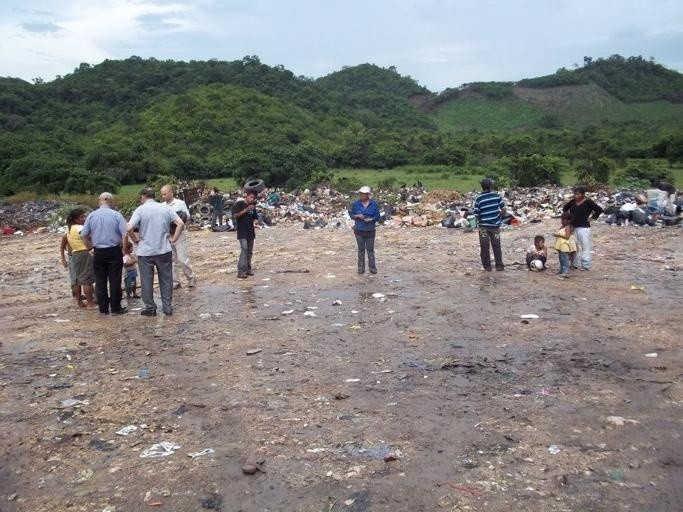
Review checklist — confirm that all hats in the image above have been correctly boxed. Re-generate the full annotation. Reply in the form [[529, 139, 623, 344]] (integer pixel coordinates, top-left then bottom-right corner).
[[97, 192, 113, 201], [358, 186, 371, 194]]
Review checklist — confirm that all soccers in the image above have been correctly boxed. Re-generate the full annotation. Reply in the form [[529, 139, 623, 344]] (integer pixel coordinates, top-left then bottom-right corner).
[[530, 259, 543, 272]]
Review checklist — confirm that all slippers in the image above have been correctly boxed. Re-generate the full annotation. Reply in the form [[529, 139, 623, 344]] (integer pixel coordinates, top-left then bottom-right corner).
[[86, 304, 100, 310]]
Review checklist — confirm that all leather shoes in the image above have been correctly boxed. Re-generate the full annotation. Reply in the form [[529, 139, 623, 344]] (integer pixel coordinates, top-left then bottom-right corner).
[[141, 309, 156, 317], [163, 309, 173, 315]]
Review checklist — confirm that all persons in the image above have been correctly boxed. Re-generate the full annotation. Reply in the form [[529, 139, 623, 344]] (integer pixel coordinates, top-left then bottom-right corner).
[[231, 190, 258, 278], [348, 186, 380, 274], [564, 187, 602, 271], [552, 212, 576, 278], [525, 236, 549, 271], [60, 185, 195, 316], [473, 179, 506, 271], [209, 188, 224, 229]]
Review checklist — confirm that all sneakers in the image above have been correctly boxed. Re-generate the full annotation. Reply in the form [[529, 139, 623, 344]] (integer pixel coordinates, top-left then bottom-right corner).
[[111, 307, 128, 316], [173, 281, 180, 289], [238, 271, 255, 278], [99, 308, 109, 314], [189, 276, 198, 287], [555, 271, 570, 278], [358, 270, 364, 274], [72, 295, 86, 300], [126, 295, 130, 299], [370, 270, 377, 274], [580, 267, 587, 271], [133, 295, 141, 298]]
